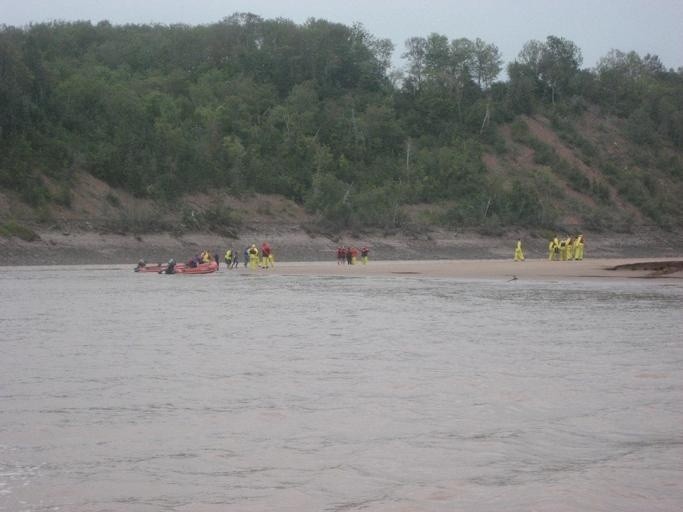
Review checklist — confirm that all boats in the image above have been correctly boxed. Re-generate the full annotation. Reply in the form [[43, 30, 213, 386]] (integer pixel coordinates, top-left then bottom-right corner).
[[135, 260, 218, 274]]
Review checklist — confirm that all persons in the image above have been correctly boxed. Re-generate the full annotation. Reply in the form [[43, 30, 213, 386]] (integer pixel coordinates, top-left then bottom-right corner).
[[513, 237, 525, 263], [189, 241, 274, 271], [548, 233, 585, 261], [334, 245, 370, 266]]
